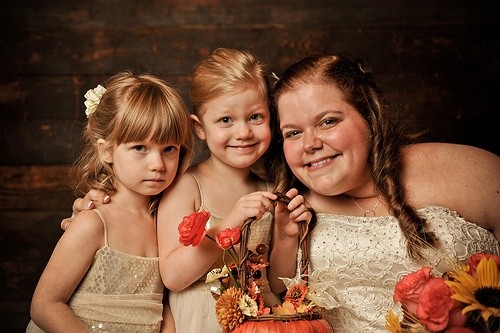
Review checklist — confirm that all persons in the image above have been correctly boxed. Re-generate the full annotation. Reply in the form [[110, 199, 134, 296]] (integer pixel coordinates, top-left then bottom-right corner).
[[25, 70, 196, 333], [60, 54, 500, 333], [156, 47, 313, 333]]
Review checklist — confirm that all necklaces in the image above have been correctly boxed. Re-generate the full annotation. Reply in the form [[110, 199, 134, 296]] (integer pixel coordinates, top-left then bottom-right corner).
[[350, 194, 382, 217]]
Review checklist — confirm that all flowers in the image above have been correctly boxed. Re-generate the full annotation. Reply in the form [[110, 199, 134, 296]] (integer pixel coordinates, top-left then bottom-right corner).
[[386, 251, 500, 333], [178, 210, 322, 332], [84, 85, 107, 119]]
